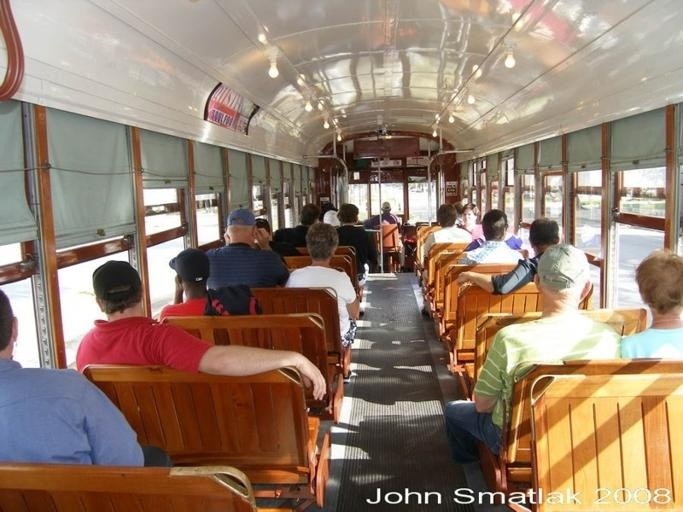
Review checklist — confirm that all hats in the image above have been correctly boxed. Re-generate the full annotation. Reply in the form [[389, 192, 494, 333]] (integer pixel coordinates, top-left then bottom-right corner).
[[169, 248, 209, 281], [227, 209, 256, 225], [381, 201, 391, 211], [93, 260, 141, 301], [537, 244, 589, 289]]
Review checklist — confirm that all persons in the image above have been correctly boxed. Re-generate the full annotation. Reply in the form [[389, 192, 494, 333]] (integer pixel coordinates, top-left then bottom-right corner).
[[200, 210, 287, 292], [443, 243, 621, 464], [249, 189, 482, 254], [75, 260, 327, 400], [0, 288, 175, 466], [284, 222, 364, 386], [450, 200, 532, 271], [161, 247, 262, 316], [455, 218, 577, 296], [621, 247, 682, 363]]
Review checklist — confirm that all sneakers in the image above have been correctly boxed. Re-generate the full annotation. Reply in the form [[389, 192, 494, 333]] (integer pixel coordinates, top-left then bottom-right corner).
[[343, 370, 352, 384]]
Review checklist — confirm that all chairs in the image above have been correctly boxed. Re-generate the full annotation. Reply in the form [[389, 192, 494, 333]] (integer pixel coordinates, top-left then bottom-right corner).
[[377, 224, 400, 271]]
